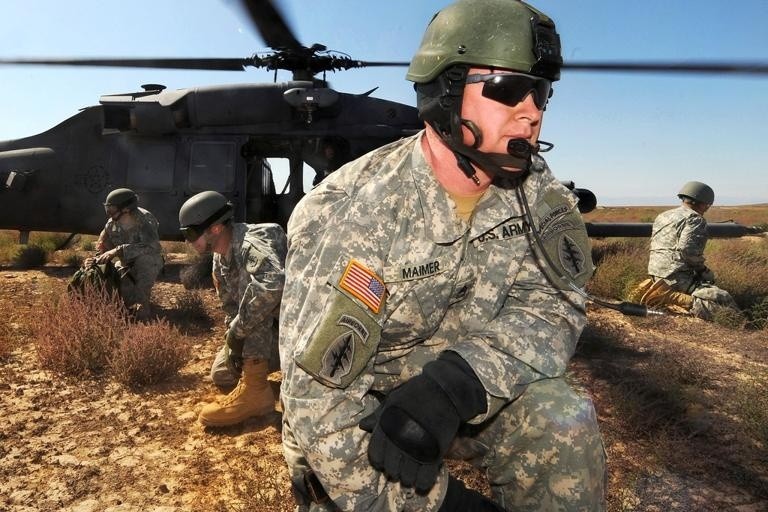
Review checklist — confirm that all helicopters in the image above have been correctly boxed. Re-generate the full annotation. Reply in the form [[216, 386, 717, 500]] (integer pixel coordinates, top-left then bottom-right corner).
[[0, 0, 768, 251]]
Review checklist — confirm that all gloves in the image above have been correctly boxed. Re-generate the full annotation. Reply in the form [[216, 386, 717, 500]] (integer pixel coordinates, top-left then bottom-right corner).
[[226, 330, 242, 352], [359, 351, 487, 496], [438, 474, 505, 512]]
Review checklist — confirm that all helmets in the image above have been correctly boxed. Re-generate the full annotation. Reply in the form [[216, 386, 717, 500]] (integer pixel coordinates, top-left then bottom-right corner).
[[404, 0, 564, 84], [179, 192, 233, 242], [679, 182, 715, 205], [104, 188, 138, 218]]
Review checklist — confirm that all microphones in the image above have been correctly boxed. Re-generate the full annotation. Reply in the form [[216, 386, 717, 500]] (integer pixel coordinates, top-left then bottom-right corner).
[[507, 138, 553, 158]]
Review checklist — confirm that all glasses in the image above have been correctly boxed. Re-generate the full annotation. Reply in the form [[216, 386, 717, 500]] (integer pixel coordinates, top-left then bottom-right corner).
[[469, 72, 552, 109], [181, 202, 229, 241]]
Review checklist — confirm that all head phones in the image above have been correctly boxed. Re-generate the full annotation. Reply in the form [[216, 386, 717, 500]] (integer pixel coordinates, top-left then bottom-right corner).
[[416, 63, 455, 121]]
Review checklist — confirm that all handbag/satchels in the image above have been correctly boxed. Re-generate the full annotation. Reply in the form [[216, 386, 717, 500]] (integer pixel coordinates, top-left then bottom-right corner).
[[66, 260, 119, 298]]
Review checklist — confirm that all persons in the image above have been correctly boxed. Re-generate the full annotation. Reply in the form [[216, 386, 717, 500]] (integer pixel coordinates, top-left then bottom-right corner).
[[276, 1, 610, 510], [312, 139, 347, 186], [178, 188, 288, 428], [84, 187, 165, 324], [626, 179, 745, 328]]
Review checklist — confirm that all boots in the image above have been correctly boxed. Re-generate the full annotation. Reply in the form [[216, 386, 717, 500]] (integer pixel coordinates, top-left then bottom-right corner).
[[198, 357, 275, 427], [628, 278, 694, 312]]
